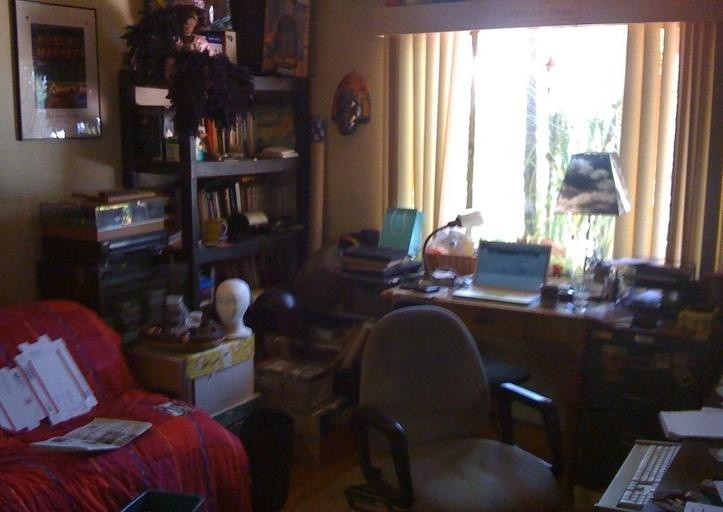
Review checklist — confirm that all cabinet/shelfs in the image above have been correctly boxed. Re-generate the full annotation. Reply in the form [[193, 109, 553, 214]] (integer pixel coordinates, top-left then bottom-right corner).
[[581, 317, 720, 478], [117, 71, 311, 327], [38, 233, 176, 337]]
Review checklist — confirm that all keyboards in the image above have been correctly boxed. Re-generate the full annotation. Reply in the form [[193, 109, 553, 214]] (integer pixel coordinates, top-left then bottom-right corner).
[[617, 445, 682, 510]]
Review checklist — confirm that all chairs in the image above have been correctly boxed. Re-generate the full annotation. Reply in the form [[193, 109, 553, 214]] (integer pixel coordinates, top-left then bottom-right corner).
[[344, 303, 567, 510]]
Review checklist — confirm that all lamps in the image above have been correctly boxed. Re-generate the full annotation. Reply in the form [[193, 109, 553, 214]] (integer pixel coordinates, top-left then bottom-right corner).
[[552, 152, 632, 302], [422, 207, 483, 280]]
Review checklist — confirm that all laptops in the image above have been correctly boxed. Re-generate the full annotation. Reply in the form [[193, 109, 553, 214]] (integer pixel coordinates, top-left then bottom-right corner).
[[452, 240, 552, 305]]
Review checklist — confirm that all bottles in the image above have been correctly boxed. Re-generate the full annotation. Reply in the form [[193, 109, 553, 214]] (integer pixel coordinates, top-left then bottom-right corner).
[[196, 125, 207, 163], [199, 277, 214, 309]]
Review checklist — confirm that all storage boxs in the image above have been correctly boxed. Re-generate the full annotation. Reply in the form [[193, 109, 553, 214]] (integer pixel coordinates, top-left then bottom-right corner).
[[253, 353, 337, 412], [259, 396, 342, 465], [213, 392, 263, 442], [132, 331, 255, 413]]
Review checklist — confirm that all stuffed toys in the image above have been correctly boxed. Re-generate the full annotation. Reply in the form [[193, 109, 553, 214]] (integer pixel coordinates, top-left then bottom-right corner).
[[160, 1, 216, 88]]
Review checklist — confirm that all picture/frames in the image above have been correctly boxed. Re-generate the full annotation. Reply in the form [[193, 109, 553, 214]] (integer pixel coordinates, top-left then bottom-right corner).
[[8, 0, 103, 140]]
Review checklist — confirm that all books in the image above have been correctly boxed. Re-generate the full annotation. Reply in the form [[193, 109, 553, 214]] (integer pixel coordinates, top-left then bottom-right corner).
[[657, 409, 723, 441]]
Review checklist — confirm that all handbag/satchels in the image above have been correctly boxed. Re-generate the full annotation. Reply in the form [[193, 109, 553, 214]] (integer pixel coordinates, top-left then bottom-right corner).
[[373, 204, 424, 260]]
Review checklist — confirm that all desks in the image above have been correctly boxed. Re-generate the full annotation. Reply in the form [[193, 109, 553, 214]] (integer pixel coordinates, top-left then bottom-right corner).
[[381, 274, 599, 454], [594, 374, 723, 512]]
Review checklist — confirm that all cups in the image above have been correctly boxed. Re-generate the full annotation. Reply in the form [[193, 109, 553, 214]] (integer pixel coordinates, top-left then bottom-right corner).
[[540, 285, 559, 310], [201, 217, 229, 249]]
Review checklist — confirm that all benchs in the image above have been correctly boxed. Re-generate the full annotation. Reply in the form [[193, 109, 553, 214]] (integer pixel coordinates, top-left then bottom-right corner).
[[0, 299, 250, 512]]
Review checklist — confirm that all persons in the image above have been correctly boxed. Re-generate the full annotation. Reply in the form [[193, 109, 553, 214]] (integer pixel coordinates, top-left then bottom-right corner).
[[139, 107, 299, 304], [213, 278, 254, 342]]
